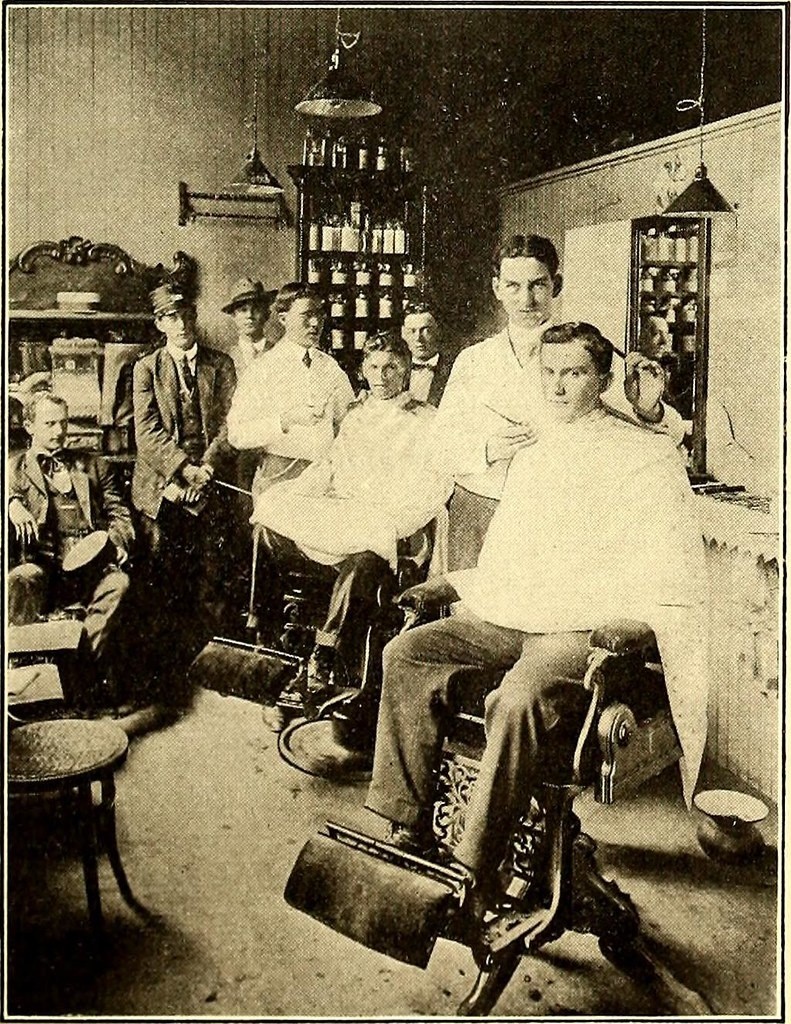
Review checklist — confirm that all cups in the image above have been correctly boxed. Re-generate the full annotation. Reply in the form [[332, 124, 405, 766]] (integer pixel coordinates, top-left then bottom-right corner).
[[353, 330, 368, 350], [330, 328, 345, 350]]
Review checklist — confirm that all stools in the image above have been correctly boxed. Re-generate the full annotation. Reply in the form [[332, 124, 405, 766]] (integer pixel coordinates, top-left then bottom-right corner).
[[9, 721, 151, 922]]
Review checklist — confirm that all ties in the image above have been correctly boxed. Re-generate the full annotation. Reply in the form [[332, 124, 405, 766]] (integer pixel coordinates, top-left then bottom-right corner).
[[302, 351, 312, 368], [180, 354, 195, 392]]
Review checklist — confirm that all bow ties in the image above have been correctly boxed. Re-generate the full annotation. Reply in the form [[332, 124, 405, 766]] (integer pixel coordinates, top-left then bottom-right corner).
[[38, 449, 65, 474], [410, 362, 436, 372]]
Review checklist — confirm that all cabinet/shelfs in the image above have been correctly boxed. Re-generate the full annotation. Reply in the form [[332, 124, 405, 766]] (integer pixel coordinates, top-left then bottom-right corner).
[[287, 167, 433, 394]]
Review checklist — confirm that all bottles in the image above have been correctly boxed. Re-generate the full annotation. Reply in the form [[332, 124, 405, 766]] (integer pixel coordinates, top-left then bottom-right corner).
[[311, 292, 426, 320], [630, 222, 698, 477], [302, 260, 420, 289], [306, 188, 420, 254], [301, 126, 416, 174]]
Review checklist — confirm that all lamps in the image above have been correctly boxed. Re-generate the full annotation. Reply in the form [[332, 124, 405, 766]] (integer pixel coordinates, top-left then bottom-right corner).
[[296, 6, 381, 118], [230, 11, 284, 195], [663, 6, 736, 219]]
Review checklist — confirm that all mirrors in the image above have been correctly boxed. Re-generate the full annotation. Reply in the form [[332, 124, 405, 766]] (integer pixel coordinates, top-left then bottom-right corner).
[[626, 215, 710, 475]]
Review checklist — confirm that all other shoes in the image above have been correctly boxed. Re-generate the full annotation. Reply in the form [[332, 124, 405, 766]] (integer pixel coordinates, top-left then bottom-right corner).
[[444, 858, 490, 904], [308, 649, 334, 685], [388, 826, 442, 864]]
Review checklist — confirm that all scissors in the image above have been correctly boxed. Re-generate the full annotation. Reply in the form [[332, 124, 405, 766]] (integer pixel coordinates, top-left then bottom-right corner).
[[309, 386, 338, 418], [486, 405, 530, 436]]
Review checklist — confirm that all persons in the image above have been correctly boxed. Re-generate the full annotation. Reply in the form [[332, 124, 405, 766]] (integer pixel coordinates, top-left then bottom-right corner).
[[129, 284, 237, 560], [641, 315, 680, 402], [432, 232, 683, 623], [365, 320, 712, 912], [7, 393, 138, 700], [220, 277, 451, 732]]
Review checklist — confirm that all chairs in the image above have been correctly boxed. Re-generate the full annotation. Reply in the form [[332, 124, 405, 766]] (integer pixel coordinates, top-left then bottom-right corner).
[[188, 485, 458, 736], [284, 567, 716, 1016]]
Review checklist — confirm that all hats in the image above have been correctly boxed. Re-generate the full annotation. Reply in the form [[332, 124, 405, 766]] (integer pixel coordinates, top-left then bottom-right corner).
[[147, 283, 189, 319], [221, 274, 278, 316], [60, 530, 120, 591]]
